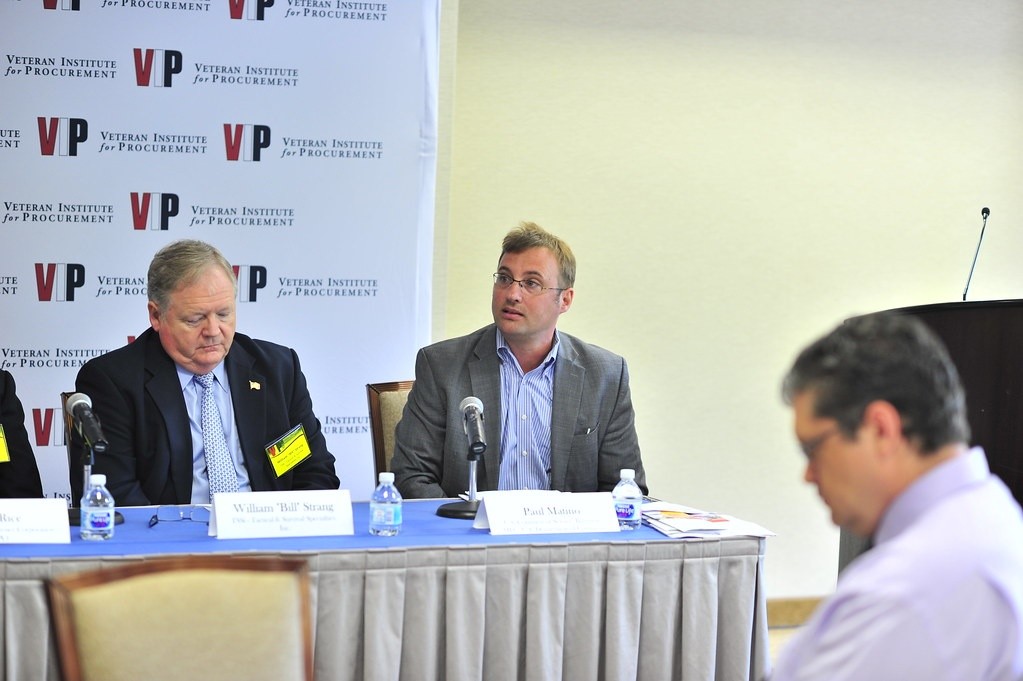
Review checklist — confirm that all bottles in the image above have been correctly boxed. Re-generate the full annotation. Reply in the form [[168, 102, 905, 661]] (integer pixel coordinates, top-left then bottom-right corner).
[[368, 472, 403, 536], [611, 468, 643, 530], [80, 474, 115, 541]]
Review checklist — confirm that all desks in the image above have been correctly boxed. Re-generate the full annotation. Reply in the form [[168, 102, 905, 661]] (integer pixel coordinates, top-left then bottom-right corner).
[[1, 497, 772, 681]]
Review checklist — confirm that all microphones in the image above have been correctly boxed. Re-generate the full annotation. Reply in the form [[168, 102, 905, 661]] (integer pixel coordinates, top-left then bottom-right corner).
[[66, 392, 109, 454], [460, 396, 487, 455], [964, 207, 990, 301]]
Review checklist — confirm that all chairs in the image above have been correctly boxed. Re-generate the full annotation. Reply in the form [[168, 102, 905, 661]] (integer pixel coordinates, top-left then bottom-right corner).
[[367, 380, 415, 492], [43, 552, 318, 680]]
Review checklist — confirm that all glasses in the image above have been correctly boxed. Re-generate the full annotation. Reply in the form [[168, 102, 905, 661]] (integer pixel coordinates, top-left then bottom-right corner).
[[802, 427, 846, 463], [492, 273, 565, 297], [148, 504, 211, 528]]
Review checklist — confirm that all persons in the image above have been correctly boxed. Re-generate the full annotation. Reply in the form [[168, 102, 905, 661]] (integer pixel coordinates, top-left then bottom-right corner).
[[770, 315, 1023, 681], [75, 240, 341, 506], [392, 228, 648, 499], [0, 369, 46, 500]]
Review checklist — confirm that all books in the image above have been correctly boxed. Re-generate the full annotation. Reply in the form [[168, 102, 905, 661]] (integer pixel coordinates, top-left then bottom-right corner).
[[640, 499, 779, 541]]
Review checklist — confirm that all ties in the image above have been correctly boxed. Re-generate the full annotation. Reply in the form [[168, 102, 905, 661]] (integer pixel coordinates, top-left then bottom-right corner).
[[193, 371, 240, 502]]
